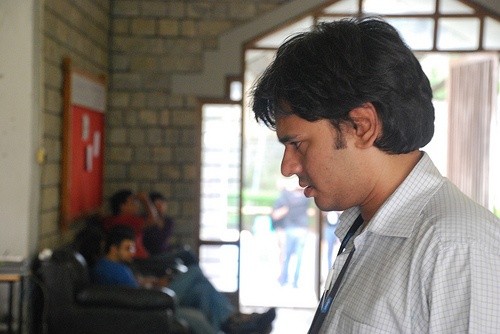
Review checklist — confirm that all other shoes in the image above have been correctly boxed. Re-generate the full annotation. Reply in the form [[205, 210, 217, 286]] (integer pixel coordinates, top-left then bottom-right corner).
[[225, 308, 276, 334]]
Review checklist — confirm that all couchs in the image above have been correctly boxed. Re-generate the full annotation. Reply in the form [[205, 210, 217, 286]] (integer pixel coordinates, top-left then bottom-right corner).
[[32, 212, 214, 334]]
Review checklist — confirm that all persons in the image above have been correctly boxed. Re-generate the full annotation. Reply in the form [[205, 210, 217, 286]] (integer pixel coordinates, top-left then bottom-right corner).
[[270, 179, 313, 290], [252, 20, 500, 333], [91, 190, 276, 334]]
